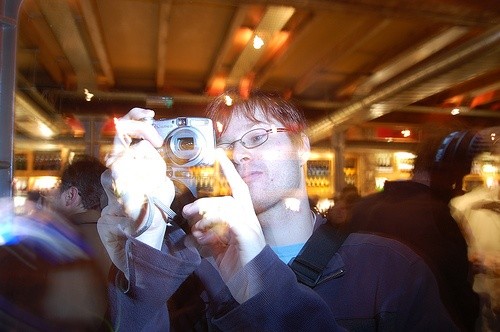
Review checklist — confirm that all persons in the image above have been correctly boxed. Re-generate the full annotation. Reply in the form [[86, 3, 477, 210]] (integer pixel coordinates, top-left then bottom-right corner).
[[308, 127, 500, 332], [97, 87, 459, 332], [0, 156, 232, 332]]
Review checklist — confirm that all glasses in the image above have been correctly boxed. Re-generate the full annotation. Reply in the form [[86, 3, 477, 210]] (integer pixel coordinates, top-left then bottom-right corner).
[[217, 126, 292, 151]]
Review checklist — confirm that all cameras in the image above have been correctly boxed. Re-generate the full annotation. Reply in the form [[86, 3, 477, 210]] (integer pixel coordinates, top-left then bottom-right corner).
[[130, 116, 217, 167]]
